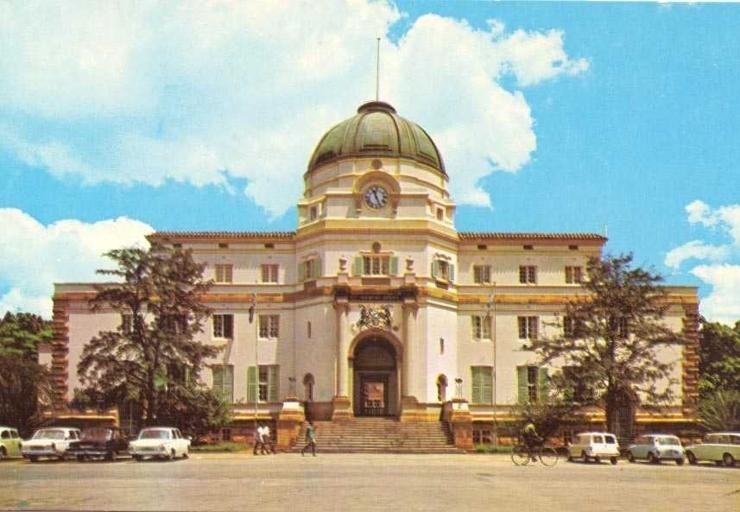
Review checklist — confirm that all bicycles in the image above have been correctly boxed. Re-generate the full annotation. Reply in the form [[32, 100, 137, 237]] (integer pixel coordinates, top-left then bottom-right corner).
[[511, 436, 559, 467]]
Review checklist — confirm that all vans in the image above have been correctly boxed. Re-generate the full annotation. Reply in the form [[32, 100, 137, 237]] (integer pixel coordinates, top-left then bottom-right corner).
[[567, 432, 621, 464]]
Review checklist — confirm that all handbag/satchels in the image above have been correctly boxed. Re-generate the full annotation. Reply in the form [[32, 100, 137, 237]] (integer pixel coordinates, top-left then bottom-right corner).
[[521, 432, 534, 446]]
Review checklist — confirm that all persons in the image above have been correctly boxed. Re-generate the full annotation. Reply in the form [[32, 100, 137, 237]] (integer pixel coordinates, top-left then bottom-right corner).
[[261, 422, 277, 455], [301, 420, 318, 457], [253, 421, 271, 455], [520, 417, 544, 462]]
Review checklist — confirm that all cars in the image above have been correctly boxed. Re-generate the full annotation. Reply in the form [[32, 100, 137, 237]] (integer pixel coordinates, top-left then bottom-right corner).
[[22, 427, 81, 460], [64, 426, 128, 463], [626, 433, 684, 465], [0, 426, 25, 460], [127, 426, 192, 460], [683, 433, 740, 467]]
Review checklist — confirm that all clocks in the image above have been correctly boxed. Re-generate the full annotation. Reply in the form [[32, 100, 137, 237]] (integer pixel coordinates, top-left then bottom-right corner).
[[364, 184, 388, 209]]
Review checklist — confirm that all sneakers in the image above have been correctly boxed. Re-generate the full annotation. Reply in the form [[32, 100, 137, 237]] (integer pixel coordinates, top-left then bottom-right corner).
[[531, 456, 537, 462]]
